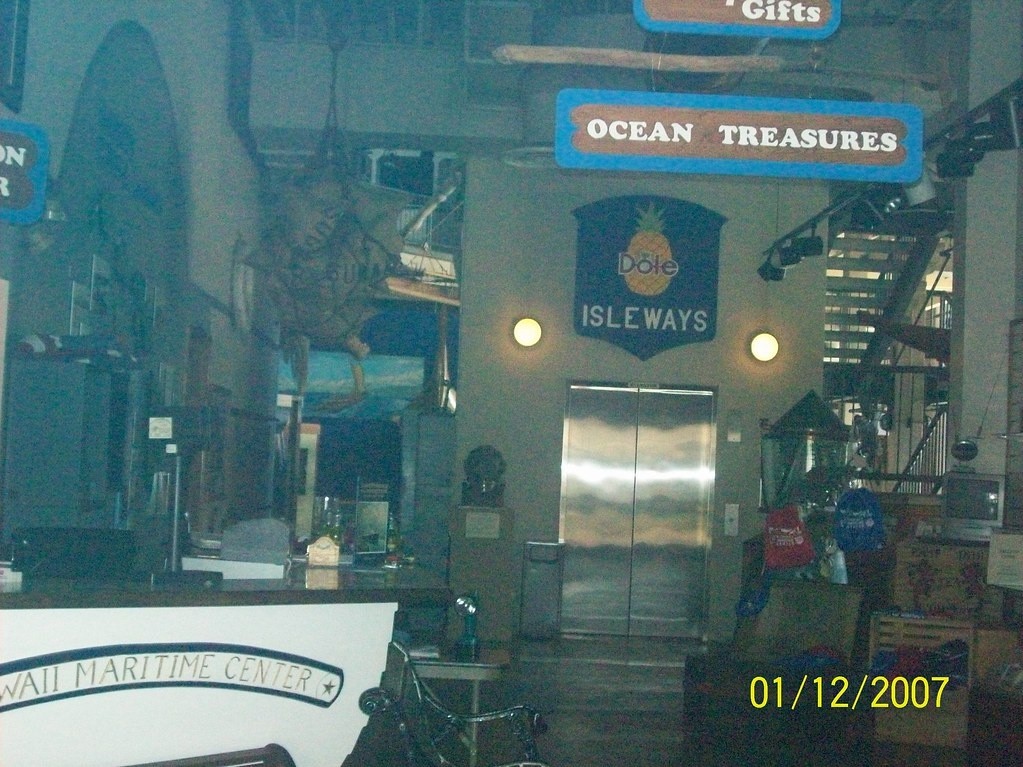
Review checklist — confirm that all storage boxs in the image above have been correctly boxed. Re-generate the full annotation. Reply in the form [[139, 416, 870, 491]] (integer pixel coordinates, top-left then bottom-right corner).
[[884, 540, 1003, 623], [682, 655, 847, 761], [867, 609, 976, 752], [868, 491, 948, 544], [731, 577, 866, 670], [974, 612, 1023, 695]]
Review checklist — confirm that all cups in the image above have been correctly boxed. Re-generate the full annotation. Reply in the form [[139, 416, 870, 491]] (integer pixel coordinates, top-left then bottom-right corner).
[[312, 497, 343, 546]]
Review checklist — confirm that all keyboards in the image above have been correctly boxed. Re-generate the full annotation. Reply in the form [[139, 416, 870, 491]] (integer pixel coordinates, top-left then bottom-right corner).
[[922, 536, 991, 548]]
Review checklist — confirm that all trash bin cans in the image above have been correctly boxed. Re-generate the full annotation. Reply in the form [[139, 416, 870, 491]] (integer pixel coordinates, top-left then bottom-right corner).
[[519, 539, 568, 644]]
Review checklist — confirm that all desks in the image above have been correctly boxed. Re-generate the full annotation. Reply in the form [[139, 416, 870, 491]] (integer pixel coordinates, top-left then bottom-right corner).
[[407, 644, 512, 767]]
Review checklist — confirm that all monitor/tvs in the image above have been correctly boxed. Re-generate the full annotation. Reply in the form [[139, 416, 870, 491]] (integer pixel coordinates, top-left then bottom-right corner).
[[941, 471, 1005, 541]]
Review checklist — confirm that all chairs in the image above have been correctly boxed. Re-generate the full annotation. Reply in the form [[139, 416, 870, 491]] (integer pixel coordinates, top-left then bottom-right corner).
[[11, 527, 139, 582], [339, 644, 546, 767]]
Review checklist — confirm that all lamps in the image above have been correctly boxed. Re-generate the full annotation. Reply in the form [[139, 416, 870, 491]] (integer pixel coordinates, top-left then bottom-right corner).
[[757, 250, 786, 282], [858, 194, 889, 226], [936, 154, 975, 178], [454, 589, 480, 649], [945, 134, 984, 165], [777, 246, 808, 267], [869, 185, 902, 214], [845, 201, 871, 233], [965, 122, 992, 151], [791, 224, 823, 256], [990, 95, 1023, 150]]
